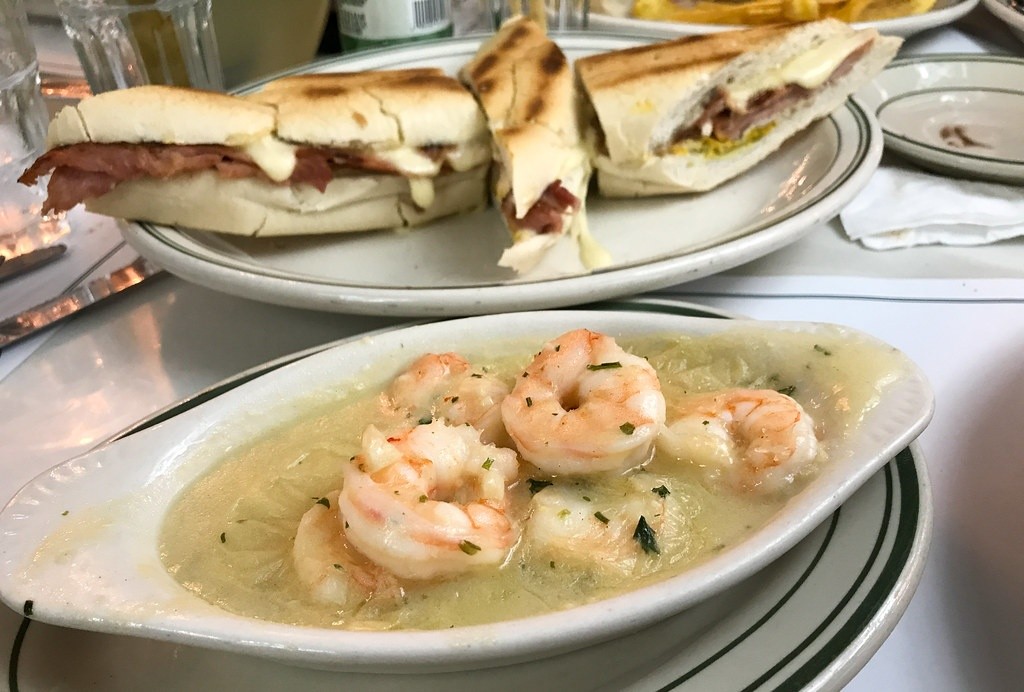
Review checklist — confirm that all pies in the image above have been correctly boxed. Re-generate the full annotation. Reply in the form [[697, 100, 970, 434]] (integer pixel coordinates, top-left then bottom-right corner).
[[13, 15, 905, 276]]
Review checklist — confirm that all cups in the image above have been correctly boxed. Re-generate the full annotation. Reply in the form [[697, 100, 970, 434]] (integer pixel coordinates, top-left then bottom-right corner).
[[56, 0, 226, 97], [336, 0, 452, 51], [0, 0, 67, 257]]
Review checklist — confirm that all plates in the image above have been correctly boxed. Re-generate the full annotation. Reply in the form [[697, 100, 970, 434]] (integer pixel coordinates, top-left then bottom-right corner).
[[547, 0, 978, 41], [0, 299, 933, 692], [120, 30, 884, 317], [0, 312, 933, 664], [854, 52, 1024, 186]]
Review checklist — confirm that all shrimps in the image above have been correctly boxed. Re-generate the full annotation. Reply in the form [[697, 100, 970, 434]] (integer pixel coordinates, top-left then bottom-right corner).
[[291, 329, 827, 608]]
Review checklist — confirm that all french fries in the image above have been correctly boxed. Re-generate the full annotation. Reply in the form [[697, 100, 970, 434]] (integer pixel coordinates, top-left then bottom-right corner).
[[627, 0, 935, 28]]
